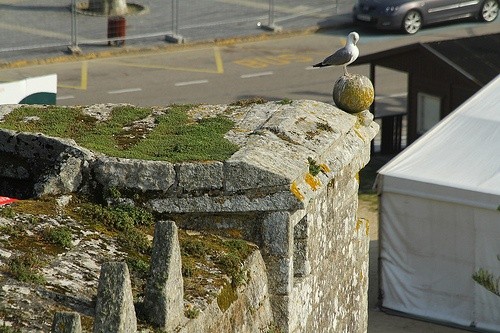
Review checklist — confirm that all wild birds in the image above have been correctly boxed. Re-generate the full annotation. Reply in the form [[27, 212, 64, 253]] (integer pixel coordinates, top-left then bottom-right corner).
[[313, 31, 360, 76]]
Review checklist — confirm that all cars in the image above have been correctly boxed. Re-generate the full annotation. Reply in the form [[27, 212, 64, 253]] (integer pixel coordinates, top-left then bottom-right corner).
[[352, 0, 500, 33]]
